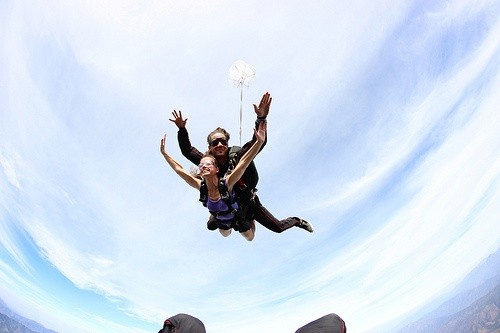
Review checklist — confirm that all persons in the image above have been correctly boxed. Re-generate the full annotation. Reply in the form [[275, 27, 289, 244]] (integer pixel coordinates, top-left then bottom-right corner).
[[160, 121, 266, 241], [169, 92, 314, 233]]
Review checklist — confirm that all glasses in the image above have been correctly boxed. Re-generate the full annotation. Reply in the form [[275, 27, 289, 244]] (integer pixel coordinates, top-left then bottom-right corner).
[[209, 138, 228, 147], [198, 160, 215, 167]]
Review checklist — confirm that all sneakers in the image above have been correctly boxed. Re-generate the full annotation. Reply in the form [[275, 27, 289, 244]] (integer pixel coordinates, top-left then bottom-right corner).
[[294, 217, 313, 232]]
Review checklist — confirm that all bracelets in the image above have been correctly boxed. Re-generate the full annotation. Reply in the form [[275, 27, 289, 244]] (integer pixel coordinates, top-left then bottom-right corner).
[[257, 116, 266, 120]]
